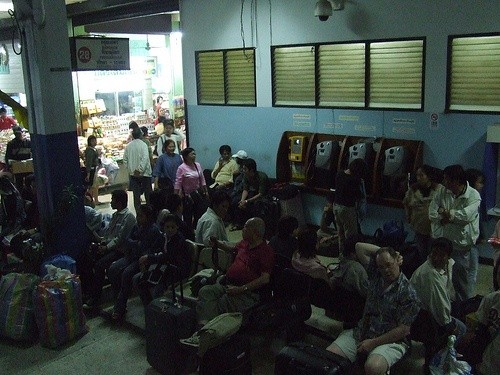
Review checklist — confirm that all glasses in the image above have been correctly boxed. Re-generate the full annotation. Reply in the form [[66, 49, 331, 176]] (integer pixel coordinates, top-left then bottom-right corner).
[[376, 260, 398, 271]]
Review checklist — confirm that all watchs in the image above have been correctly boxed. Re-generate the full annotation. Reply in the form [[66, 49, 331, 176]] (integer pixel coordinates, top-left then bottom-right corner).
[[443, 209, 448, 215], [243, 285, 247, 291]]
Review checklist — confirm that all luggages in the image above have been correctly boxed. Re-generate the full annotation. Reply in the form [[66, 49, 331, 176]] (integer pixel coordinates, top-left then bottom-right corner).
[[274, 341, 352, 375], [198, 333, 252, 375], [145, 263, 198, 375]]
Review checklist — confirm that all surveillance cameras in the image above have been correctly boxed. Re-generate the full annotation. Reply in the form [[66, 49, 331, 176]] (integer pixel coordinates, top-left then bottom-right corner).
[[314, 0, 332, 22]]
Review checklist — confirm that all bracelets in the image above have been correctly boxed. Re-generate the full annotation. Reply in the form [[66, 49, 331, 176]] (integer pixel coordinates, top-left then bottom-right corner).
[[246, 200, 249, 204]]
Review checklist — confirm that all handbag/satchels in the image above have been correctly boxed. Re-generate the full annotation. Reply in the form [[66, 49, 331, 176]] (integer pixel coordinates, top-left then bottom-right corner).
[[197, 312, 243, 358], [138, 256, 178, 298], [184, 186, 207, 211], [189, 236, 221, 297]]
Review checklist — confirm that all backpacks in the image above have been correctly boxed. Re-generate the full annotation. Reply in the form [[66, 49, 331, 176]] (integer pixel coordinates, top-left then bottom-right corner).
[[328, 260, 369, 300], [273, 181, 299, 200], [374, 221, 405, 248]]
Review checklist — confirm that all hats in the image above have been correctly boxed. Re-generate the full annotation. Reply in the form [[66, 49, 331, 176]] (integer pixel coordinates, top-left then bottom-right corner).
[[232, 150, 248, 160], [13, 127, 22, 135]]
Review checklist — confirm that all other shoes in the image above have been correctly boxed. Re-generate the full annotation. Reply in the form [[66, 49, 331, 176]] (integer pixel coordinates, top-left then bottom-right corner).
[[82, 299, 95, 309], [229, 225, 242, 231], [112, 311, 122, 321]]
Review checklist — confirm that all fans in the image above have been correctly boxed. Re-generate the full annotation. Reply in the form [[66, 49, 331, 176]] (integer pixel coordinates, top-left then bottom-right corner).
[[135, 34, 161, 50]]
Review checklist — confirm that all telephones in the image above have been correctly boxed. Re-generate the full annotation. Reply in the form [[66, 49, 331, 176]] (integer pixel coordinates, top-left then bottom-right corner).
[[348, 143, 368, 175], [383, 146, 405, 177], [316, 141, 334, 170], [288, 136, 305, 162]]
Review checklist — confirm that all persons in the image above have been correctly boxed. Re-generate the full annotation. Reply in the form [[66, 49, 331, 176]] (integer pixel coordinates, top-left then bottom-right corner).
[[488, 219, 500, 291], [474, 256, 500, 375], [409, 237, 467, 360], [124, 94, 186, 210], [0, 108, 40, 260], [325, 242, 420, 375], [179, 218, 275, 347], [404, 165, 488, 261], [84, 135, 105, 205], [315, 193, 338, 255], [332, 159, 367, 252], [133, 215, 188, 299], [172, 144, 271, 233], [108, 205, 160, 323], [428, 165, 481, 300], [82, 189, 136, 310], [195, 191, 229, 247], [84, 189, 103, 238], [269, 215, 370, 291]]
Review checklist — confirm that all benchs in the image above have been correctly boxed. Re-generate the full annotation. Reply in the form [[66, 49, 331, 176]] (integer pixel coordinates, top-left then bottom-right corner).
[[202, 169, 281, 225], [174, 239, 412, 375]]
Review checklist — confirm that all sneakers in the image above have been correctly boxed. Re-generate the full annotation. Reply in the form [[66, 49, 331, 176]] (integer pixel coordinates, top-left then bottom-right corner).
[[179, 331, 200, 347]]
